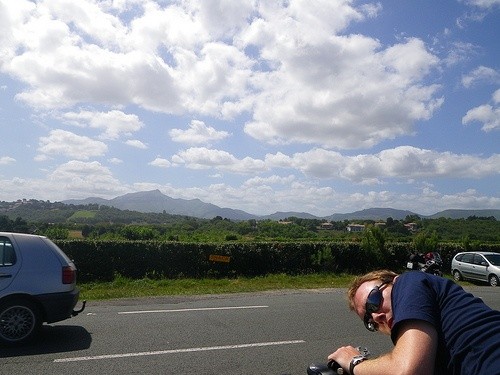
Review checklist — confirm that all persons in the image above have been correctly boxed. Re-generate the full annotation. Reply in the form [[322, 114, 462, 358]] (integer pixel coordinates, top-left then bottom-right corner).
[[327, 269, 500, 375]]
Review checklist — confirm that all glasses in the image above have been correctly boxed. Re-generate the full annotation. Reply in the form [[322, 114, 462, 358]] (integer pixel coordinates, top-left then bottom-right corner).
[[364, 281, 390, 331]]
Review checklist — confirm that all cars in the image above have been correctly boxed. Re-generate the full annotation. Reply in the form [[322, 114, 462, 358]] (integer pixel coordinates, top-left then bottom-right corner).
[[451, 251, 500, 287], [1, 231, 79, 342]]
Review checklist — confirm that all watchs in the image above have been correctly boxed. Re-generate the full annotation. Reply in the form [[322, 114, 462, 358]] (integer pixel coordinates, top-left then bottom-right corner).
[[346, 355, 367, 373]]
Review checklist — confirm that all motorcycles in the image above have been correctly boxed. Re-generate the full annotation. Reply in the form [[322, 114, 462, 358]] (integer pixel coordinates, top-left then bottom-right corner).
[[405, 250, 443, 277]]
